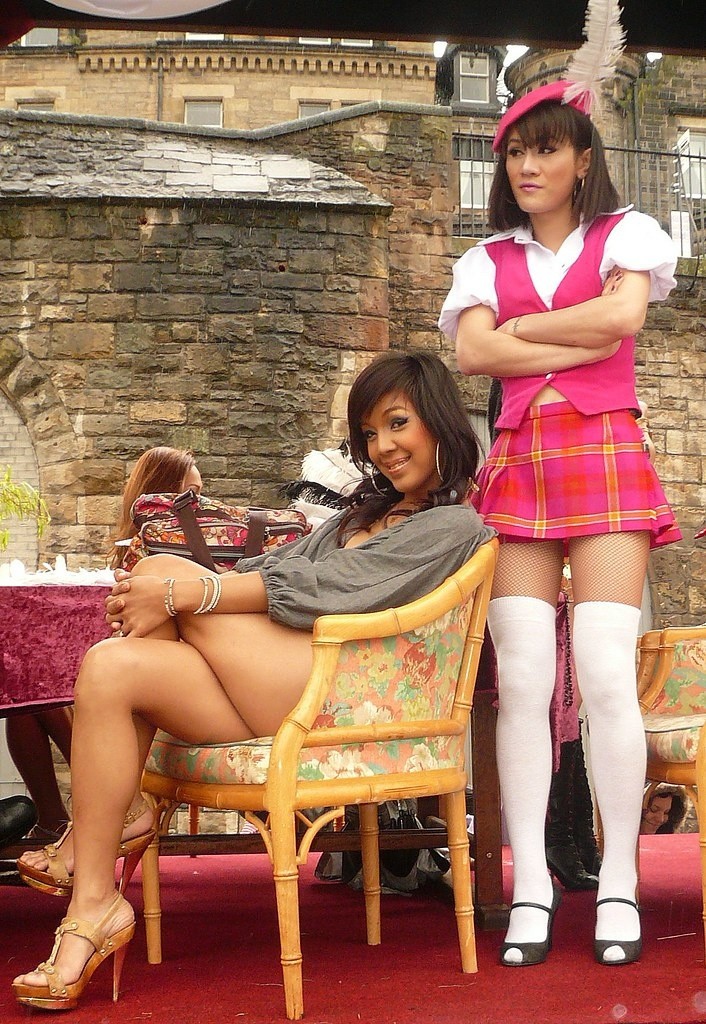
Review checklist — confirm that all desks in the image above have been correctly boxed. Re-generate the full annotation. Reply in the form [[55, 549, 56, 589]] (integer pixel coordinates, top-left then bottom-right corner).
[[0, 584, 118, 719]]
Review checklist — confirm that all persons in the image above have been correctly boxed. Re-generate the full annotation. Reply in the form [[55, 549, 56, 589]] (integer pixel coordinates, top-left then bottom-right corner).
[[6, 706, 72, 844], [12, 349, 492, 1011], [544, 592, 603, 892], [637, 778, 689, 835], [435, 79, 682, 967], [109, 446, 204, 569]]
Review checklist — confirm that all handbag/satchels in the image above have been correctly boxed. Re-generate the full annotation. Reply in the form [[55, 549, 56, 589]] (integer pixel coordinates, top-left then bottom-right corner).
[[120, 492, 311, 572]]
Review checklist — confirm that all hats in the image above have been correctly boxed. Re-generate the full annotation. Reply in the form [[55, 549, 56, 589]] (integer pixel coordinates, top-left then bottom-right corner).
[[492, 81, 593, 154]]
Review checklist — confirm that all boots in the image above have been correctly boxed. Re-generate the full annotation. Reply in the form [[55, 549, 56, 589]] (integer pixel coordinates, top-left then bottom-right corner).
[[574, 740, 602, 875], [545, 739, 599, 893]]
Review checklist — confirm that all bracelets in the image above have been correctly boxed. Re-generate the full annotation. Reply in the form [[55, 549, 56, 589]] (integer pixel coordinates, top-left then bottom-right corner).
[[195, 575, 222, 614], [164, 577, 179, 615]]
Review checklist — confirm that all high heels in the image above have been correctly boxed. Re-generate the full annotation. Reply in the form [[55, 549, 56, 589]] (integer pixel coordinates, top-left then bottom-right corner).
[[16, 799, 158, 896], [12, 894, 135, 1008], [594, 898, 642, 965], [500, 880, 562, 966]]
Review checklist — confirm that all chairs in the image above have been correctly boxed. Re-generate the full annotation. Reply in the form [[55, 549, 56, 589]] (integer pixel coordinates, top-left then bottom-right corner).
[[138, 536, 500, 1021], [636, 623, 706, 931]]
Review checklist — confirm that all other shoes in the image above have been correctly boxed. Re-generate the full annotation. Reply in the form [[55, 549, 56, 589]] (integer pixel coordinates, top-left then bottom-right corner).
[[0, 795, 38, 850], [22, 820, 68, 839]]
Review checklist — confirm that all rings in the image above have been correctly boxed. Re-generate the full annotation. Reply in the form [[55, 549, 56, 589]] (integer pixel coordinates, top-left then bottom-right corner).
[[118, 628, 123, 638]]
